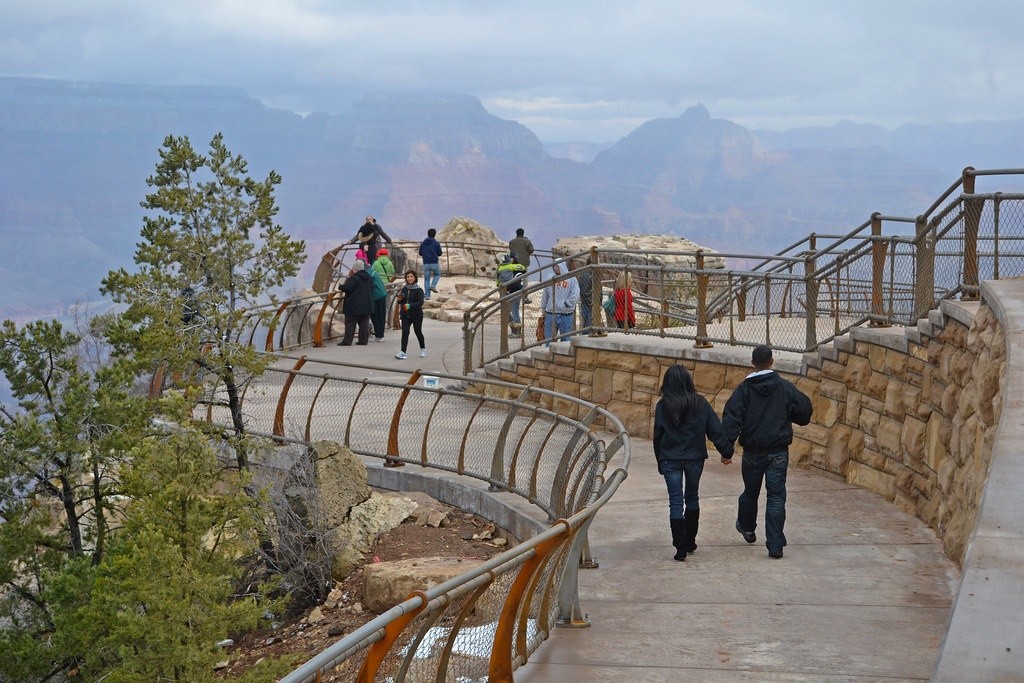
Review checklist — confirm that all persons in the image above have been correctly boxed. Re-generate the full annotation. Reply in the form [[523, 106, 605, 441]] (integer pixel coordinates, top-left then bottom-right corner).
[[495, 253, 532, 338], [348, 215, 391, 268], [419, 229, 442, 300], [337, 242, 395, 346], [653, 364, 734, 562], [165, 288, 204, 383], [394, 269, 427, 358], [541, 246, 635, 348], [509, 228, 534, 303], [720, 344, 813, 558]]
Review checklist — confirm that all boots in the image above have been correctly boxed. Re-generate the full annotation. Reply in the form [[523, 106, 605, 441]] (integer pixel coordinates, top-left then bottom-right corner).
[[670, 516, 687, 559], [513, 323, 523, 337], [684, 508, 699, 552], [508, 323, 516, 337]]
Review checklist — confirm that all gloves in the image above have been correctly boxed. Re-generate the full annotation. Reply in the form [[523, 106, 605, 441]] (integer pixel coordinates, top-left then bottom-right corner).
[[404, 303, 410, 310], [398, 293, 405, 300]]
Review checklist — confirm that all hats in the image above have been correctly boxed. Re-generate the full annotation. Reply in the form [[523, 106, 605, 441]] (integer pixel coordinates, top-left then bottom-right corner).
[[510, 252, 519, 259], [501, 253, 510, 261], [376, 248, 388, 256], [352, 259, 364, 270]]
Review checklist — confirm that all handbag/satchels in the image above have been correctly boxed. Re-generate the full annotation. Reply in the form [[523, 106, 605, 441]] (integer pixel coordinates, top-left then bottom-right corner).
[[537, 315, 557, 341], [603, 292, 618, 317]]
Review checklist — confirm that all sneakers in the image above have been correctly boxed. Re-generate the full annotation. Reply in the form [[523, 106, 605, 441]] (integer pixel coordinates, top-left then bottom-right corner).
[[395, 350, 407, 358], [420, 348, 427, 356]]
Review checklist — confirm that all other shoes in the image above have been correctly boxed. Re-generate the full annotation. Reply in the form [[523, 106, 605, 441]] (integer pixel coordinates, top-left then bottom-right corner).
[[370, 337, 384, 342], [736, 519, 757, 542], [766, 540, 783, 557], [525, 299, 532, 303], [430, 287, 438, 293], [337, 341, 351, 345], [355, 341, 368, 345]]
